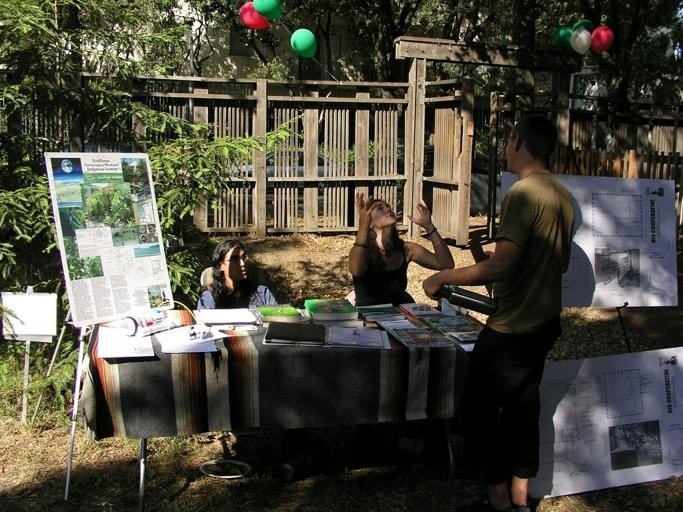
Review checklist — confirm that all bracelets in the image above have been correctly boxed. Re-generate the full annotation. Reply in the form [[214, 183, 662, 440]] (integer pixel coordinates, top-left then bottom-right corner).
[[434, 239, 442, 249], [352, 243, 368, 249], [427, 227, 437, 236]]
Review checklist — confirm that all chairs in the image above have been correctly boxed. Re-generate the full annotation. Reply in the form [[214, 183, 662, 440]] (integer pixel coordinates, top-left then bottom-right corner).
[[199, 265, 215, 293]]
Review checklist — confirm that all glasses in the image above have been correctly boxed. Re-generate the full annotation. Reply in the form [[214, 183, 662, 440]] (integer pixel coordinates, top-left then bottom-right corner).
[[225, 254, 249, 263]]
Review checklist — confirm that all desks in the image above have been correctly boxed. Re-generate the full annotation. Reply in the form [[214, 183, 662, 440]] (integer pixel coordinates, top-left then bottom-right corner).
[[83, 306, 484, 511]]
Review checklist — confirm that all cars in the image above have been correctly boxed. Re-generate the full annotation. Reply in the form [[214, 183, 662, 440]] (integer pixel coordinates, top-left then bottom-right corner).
[[406, 144, 498, 177], [347, 157, 403, 191], [232, 149, 345, 194]]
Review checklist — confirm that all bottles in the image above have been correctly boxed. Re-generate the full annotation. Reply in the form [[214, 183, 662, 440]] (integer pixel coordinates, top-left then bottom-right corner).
[[432, 283, 499, 316]]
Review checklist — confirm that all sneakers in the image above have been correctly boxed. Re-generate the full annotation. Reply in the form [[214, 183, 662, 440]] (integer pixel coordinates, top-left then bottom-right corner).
[[456, 493, 531, 512]]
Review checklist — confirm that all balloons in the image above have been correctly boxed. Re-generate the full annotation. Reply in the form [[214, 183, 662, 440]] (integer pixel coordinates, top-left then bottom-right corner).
[[239, 1, 269, 31], [253, 0, 282, 20], [290, 28, 317, 59], [552, 19, 615, 56]]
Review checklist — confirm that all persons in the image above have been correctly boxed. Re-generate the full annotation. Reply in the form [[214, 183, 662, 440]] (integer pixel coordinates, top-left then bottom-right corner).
[[348, 193, 455, 312], [422, 112, 576, 512], [196, 239, 278, 309]]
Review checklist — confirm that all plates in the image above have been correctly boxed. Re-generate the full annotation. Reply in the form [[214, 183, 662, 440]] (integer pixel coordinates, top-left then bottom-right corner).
[[199, 459, 253, 480]]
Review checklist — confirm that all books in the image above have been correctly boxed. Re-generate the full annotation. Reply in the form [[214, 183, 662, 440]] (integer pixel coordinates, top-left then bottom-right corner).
[[98, 298, 481, 359]]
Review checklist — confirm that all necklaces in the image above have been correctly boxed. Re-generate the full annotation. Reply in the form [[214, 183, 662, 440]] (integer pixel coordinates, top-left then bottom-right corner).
[[377, 246, 396, 258]]
